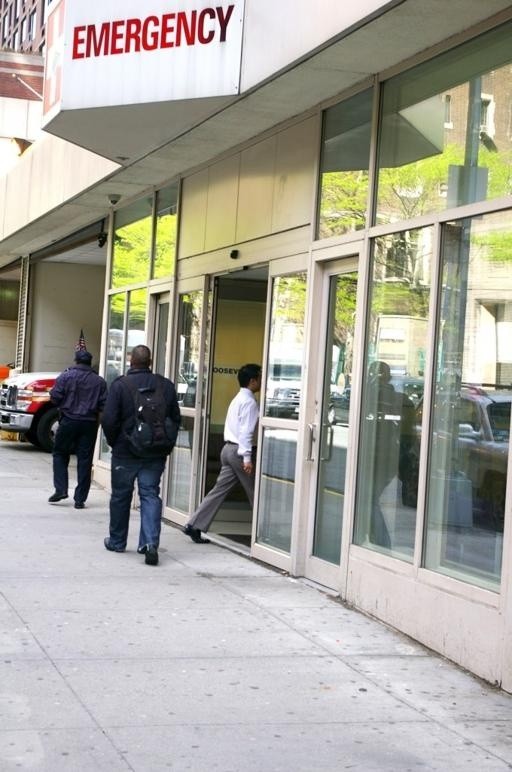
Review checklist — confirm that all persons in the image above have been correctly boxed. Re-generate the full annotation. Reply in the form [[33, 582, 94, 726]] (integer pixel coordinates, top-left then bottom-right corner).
[[43, 349, 108, 511], [97, 344, 182, 567], [364, 358, 418, 550], [183, 362, 267, 548]]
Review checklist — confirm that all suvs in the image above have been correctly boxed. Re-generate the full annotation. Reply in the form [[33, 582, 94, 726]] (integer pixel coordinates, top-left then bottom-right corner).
[[0, 363, 99, 454]]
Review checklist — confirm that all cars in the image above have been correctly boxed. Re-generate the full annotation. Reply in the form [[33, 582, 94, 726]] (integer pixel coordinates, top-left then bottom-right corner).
[[333, 376, 423, 424], [399, 383, 512, 533], [106, 329, 188, 431]]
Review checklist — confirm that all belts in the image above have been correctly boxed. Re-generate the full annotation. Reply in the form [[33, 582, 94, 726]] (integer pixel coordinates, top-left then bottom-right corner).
[[227, 440, 238, 446]]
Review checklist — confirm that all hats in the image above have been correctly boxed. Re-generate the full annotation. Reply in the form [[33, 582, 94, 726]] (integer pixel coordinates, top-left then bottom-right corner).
[[74, 350, 93, 361]]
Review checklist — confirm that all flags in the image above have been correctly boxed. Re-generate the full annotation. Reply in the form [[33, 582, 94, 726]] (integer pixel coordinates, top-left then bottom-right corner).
[[75, 330, 88, 352]]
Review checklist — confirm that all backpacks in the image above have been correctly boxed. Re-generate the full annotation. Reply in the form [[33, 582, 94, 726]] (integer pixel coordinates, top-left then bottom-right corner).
[[119, 373, 180, 461]]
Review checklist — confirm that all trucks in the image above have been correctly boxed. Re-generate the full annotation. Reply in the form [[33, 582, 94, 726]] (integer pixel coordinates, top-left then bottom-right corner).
[[265, 342, 341, 419]]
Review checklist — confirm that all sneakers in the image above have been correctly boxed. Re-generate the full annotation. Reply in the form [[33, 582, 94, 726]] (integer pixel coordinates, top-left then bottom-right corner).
[[145, 543, 159, 565], [182, 524, 210, 543], [74, 500, 85, 509], [48, 491, 69, 502], [103, 536, 125, 553]]
